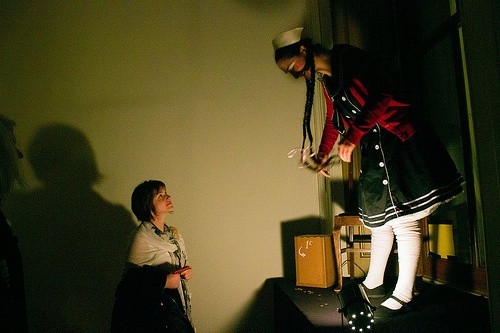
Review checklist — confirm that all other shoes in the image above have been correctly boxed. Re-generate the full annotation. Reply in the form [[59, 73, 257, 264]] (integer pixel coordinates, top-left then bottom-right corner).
[[375, 294, 418, 316], [359, 282, 385, 298]]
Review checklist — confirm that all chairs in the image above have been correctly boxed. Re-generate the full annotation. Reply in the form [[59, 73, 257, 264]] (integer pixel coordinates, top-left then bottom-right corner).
[[334, 152, 428, 293]]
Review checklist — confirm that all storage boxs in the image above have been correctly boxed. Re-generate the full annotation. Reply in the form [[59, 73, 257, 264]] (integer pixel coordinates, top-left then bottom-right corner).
[[293, 234, 336, 288]]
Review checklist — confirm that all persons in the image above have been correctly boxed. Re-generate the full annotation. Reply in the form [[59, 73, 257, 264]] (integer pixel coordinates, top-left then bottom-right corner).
[[122, 180, 197, 333], [271, 26, 468, 320]]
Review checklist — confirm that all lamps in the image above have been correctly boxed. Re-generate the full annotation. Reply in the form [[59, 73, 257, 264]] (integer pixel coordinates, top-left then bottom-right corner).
[[337, 284, 376, 333]]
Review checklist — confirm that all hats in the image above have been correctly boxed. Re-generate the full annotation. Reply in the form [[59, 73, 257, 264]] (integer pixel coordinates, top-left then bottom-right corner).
[[272, 27, 303, 51]]
